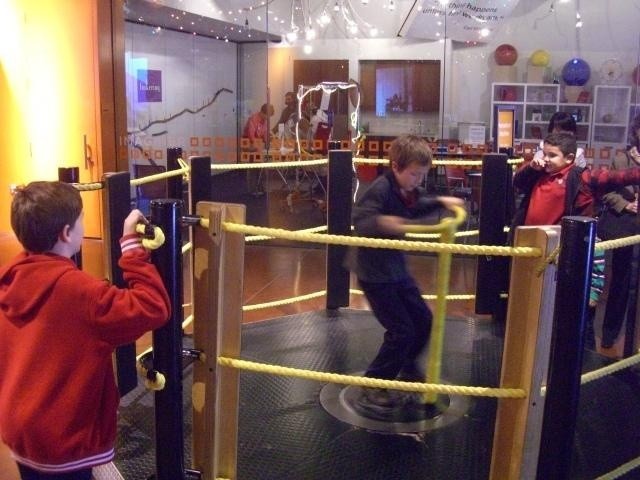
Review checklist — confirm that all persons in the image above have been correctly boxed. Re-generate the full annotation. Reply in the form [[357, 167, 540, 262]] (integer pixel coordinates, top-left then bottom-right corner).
[[344, 133, 466, 407], [272, 91, 298, 134], [596, 114, 640, 357], [532, 111, 587, 168], [0, 180, 173, 480], [243, 102, 275, 197], [507, 132, 640, 245]]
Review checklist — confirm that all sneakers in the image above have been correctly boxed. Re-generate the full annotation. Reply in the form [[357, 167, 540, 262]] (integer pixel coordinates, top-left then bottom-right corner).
[[360, 387, 425, 407]]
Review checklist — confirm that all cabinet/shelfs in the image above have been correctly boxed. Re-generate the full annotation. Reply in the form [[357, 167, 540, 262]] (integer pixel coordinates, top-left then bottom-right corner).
[[489, 82, 640, 171]]
